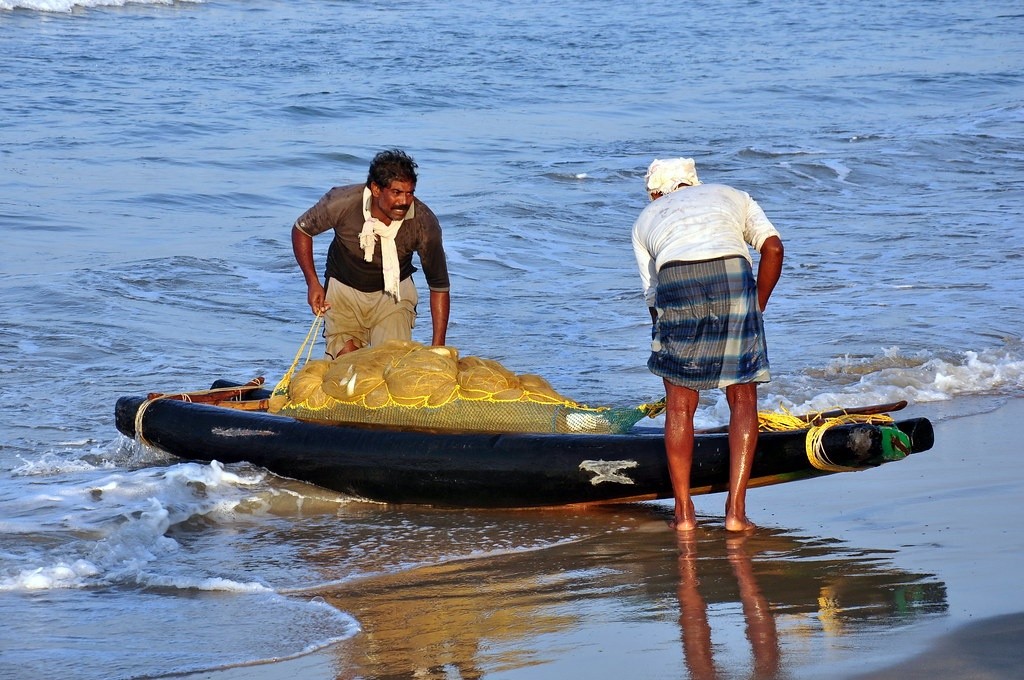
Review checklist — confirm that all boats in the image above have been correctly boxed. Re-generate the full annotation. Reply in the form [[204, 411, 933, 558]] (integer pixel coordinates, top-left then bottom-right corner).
[[111, 379, 936, 521]]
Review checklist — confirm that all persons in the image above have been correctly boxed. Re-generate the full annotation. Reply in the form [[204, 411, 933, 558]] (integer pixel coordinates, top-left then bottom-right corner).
[[630, 157, 784, 532], [291, 150, 450, 358], [677, 529, 781, 680]]
